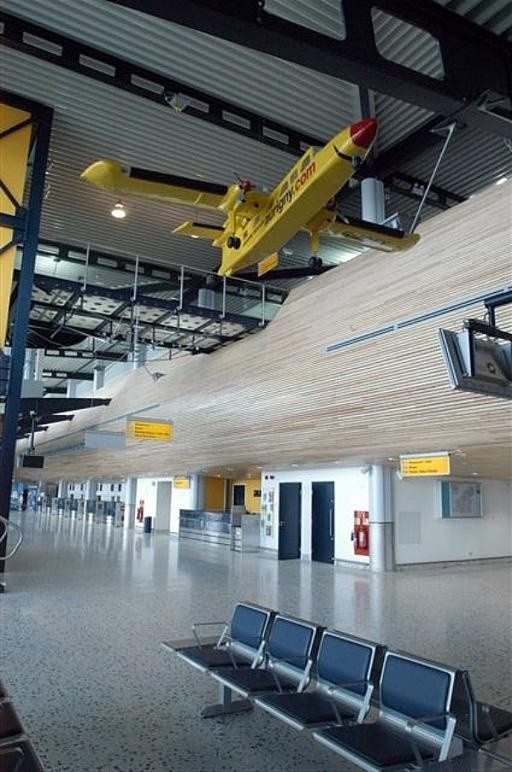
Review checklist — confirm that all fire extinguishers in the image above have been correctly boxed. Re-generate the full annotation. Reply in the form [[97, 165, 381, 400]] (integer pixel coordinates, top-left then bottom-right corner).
[[144, 518, 153, 534]]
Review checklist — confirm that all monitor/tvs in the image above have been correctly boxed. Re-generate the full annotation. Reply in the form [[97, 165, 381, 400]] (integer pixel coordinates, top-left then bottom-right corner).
[[23, 456, 44, 468], [436, 327, 512, 398]]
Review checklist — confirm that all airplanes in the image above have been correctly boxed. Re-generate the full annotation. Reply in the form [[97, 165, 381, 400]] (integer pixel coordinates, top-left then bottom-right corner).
[[78, 118, 420, 283]]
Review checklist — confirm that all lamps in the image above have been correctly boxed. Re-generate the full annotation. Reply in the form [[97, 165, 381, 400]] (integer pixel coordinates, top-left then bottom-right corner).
[[111, 200, 126, 220]]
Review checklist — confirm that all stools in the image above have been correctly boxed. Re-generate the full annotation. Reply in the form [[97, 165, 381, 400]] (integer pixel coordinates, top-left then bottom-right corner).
[[1, 679, 46, 771]]
